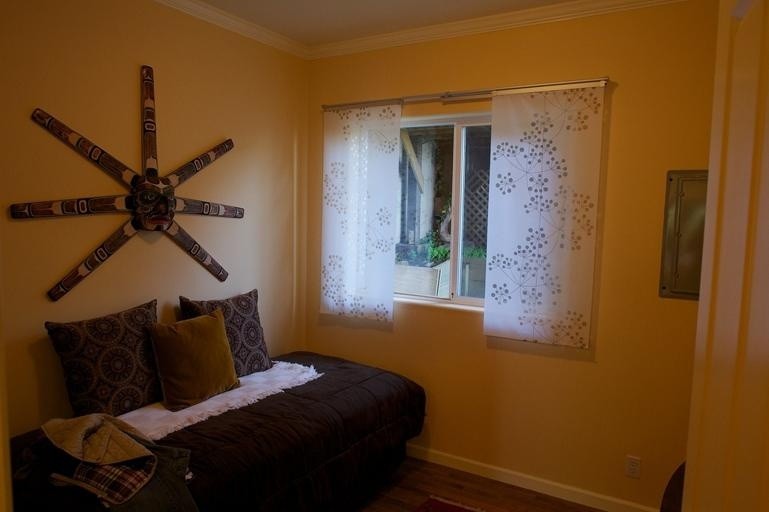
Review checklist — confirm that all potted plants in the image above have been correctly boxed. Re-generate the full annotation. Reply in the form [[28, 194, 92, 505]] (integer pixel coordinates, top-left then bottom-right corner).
[[393, 228, 486, 298]]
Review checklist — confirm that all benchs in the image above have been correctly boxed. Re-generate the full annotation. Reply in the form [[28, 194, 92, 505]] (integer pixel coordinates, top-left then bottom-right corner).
[[10, 350, 426, 511]]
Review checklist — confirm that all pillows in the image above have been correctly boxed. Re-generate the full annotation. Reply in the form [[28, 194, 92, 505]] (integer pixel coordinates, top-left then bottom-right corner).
[[44, 288, 276, 418]]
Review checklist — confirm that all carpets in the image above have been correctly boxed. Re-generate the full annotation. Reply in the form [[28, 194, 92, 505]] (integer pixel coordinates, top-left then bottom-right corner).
[[416, 493, 485, 512]]
